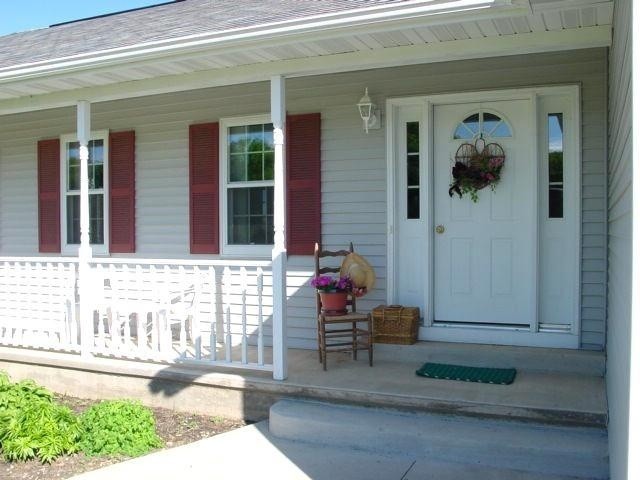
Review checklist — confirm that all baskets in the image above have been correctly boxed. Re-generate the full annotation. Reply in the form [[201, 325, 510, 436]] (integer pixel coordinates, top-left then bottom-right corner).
[[372, 306, 421, 344]]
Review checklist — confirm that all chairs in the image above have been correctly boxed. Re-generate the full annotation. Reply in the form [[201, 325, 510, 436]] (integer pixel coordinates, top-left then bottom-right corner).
[[314, 241, 373, 373]]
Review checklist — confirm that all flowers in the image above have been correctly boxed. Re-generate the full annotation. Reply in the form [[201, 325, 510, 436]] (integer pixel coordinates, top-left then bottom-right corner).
[[449, 151, 504, 199]]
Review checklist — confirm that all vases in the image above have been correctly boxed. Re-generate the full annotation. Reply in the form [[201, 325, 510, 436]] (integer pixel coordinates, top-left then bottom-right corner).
[[318, 291, 347, 315], [311, 276, 349, 292]]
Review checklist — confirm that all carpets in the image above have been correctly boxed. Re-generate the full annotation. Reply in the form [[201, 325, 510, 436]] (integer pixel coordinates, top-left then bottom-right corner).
[[415, 362, 517, 386]]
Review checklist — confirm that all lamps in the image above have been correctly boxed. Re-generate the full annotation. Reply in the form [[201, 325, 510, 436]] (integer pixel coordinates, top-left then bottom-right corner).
[[357, 88, 382, 135]]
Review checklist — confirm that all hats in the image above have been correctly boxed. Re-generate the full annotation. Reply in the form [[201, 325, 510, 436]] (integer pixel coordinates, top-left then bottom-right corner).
[[340, 253, 375, 297]]
